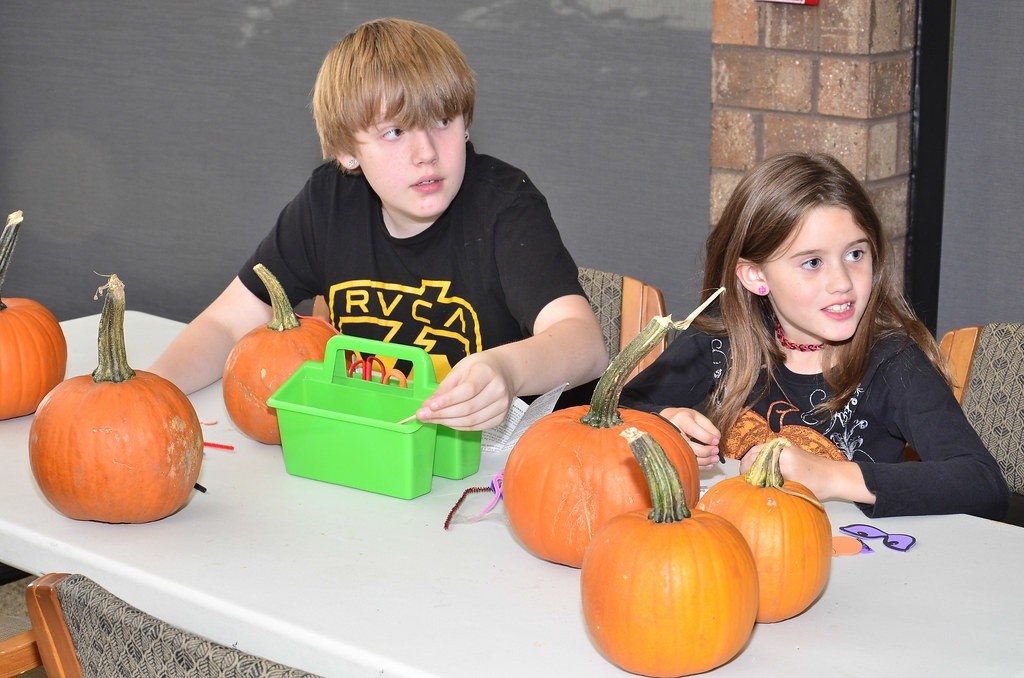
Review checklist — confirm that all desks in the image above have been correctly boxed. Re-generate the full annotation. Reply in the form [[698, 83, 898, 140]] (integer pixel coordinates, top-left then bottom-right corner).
[[0, 309, 1024, 678]]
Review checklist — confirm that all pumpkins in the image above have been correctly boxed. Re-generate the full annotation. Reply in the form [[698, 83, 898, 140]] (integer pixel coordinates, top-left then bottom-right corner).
[[502, 286, 831, 677], [0, 208, 67, 421], [29, 274, 204, 524], [223, 263, 355, 446]]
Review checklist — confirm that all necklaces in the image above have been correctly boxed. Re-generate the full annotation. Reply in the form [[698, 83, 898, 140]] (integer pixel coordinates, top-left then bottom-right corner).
[[766, 315, 830, 357]]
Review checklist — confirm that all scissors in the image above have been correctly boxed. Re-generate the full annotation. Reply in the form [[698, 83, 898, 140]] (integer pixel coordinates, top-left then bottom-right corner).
[[347, 355, 386, 384]]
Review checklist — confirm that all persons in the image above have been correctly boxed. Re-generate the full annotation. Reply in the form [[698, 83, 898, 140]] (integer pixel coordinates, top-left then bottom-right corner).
[[621, 148, 1013, 520], [140, 8, 613, 431]]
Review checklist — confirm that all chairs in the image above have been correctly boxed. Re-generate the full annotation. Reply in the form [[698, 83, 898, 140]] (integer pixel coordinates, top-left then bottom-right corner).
[[924, 321, 1023, 501], [0, 571, 326, 678], [575, 266, 669, 385]]
